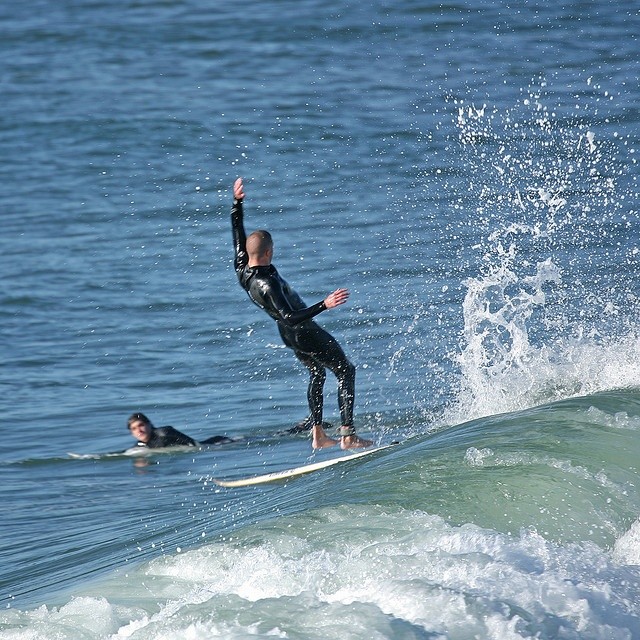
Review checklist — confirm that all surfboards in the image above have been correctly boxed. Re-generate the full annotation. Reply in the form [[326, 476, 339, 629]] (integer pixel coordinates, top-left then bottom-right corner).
[[212, 440, 405, 487]]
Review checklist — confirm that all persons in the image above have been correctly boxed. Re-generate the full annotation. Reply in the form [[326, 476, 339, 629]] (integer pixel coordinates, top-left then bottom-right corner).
[[128, 413, 332, 455], [230, 177, 387, 451]]
